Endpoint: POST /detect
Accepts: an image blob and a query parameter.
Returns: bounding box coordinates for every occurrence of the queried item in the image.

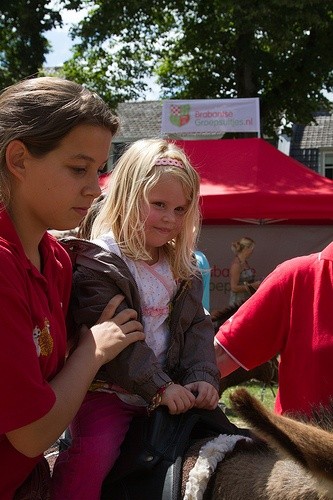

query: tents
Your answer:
[96,139,333,227]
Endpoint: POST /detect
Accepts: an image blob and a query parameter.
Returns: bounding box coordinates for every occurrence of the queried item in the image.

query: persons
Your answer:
[230,237,261,305]
[213,241,333,433]
[51,137,220,499]
[0,77,145,500]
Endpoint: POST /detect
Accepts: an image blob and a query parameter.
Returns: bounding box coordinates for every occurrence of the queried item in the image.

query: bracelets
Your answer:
[148,380,172,412]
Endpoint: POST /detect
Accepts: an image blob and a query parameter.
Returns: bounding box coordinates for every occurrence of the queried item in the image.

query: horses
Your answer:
[98,385,333,499]
